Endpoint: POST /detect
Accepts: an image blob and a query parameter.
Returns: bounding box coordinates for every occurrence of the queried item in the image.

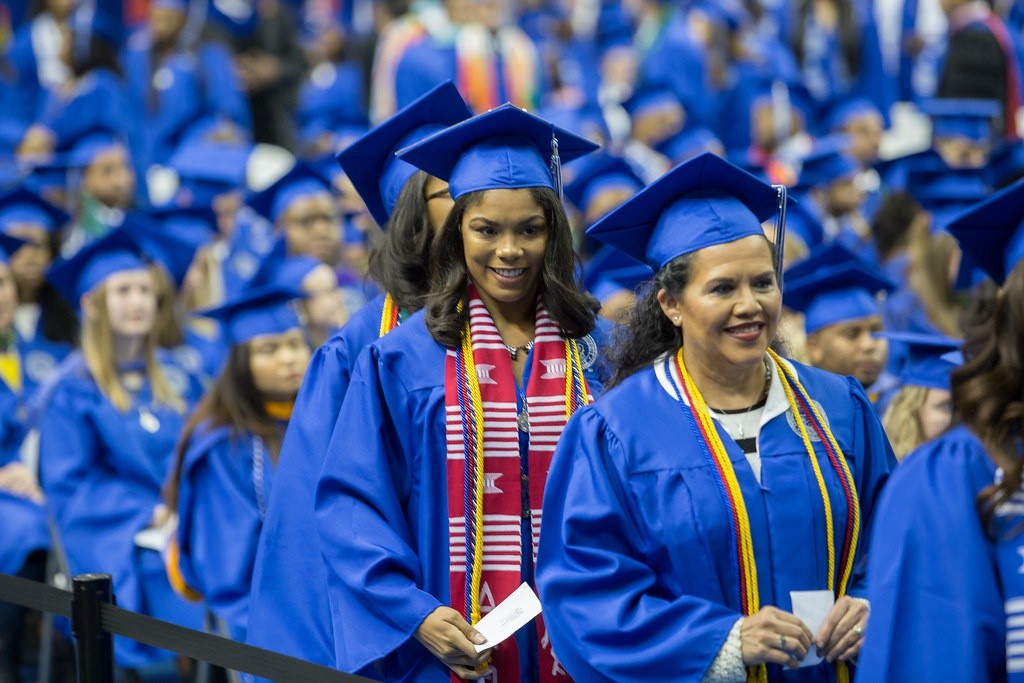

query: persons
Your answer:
[781,262,897,387]
[41,230,204,683]
[856,179,1023,683]
[534,151,897,683]
[245,160,347,261]
[870,328,969,460]
[0,0,1023,581]
[173,279,315,645]
[315,102,640,683]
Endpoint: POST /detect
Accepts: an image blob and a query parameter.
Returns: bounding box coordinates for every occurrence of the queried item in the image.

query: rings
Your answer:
[854,626,864,637]
[780,634,784,648]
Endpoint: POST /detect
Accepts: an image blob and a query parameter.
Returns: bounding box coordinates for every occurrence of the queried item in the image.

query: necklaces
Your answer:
[765,361,772,395]
[507,340,535,359]
[711,380,765,439]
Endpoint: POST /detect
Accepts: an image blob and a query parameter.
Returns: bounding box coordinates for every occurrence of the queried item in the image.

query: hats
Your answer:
[240,153,345,226]
[870,332,965,390]
[562,154,646,210]
[193,284,312,345]
[780,146,851,190]
[780,242,899,332]
[944,177,1024,287]
[585,152,797,287]
[395,103,599,204]
[45,226,151,322]
[763,192,824,258]
[246,235,325,301]
[915,97,1000,138]
[335,78,482,232]
